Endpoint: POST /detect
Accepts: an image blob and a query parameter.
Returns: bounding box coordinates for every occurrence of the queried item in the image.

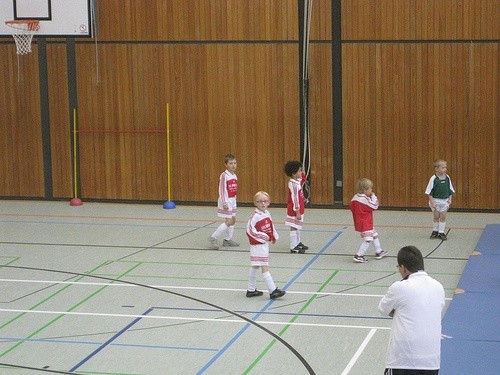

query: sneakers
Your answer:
[222,239,240,246]
[207,237,218,250]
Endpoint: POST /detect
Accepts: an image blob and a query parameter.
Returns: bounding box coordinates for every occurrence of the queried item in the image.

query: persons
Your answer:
[425,159,455,240]
[208,154,240,250]
[348,178,389,263]
[245,191,286,300]
[378,246,445,375]
[284,160,308,254]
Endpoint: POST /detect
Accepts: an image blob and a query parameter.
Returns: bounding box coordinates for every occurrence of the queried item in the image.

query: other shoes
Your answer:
[270,289,285,299]
[246,289,263,297]
[290,243,309,255]
[375,250,389,258]
[354,254,366,261]
[429,230,447,241]
